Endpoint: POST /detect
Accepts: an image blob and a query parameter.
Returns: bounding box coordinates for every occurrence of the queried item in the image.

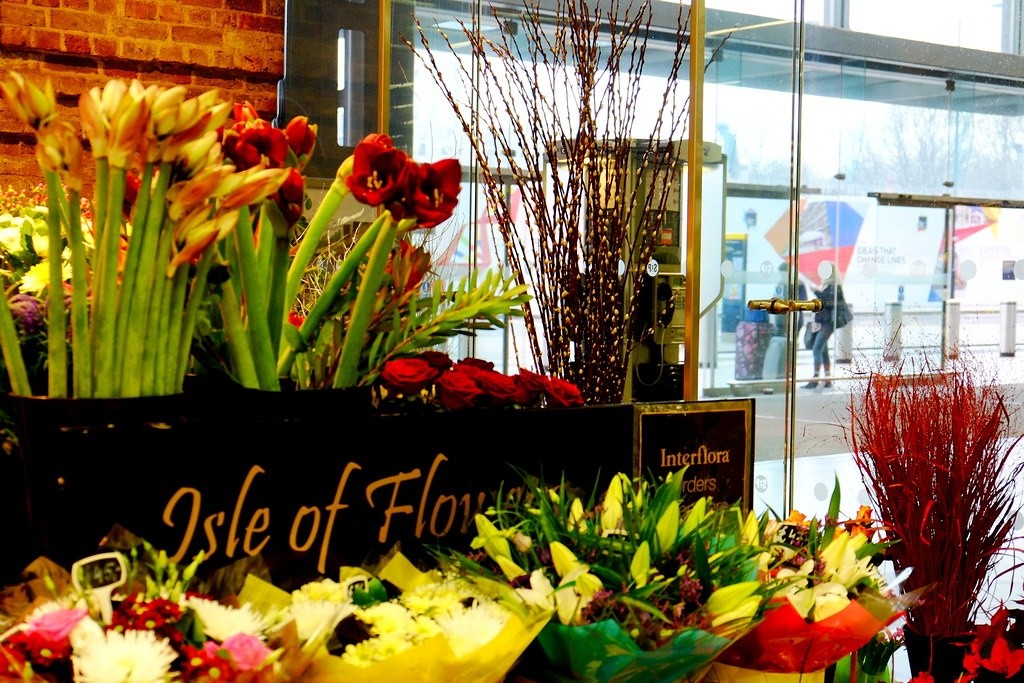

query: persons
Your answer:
[761,261,852,388]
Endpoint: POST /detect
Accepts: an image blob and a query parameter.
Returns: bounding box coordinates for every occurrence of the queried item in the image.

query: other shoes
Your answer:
[801,377,819,389]
[823,381,832,389]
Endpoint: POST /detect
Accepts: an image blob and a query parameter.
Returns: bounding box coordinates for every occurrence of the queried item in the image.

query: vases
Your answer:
[14,386,195,431]
[214,363,374,418]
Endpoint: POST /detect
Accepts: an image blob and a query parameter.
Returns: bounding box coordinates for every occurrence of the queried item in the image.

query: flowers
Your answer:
[1,1,1024,683]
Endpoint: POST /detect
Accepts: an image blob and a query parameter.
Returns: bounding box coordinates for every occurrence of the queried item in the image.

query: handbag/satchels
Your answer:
[830,285,854,328]
